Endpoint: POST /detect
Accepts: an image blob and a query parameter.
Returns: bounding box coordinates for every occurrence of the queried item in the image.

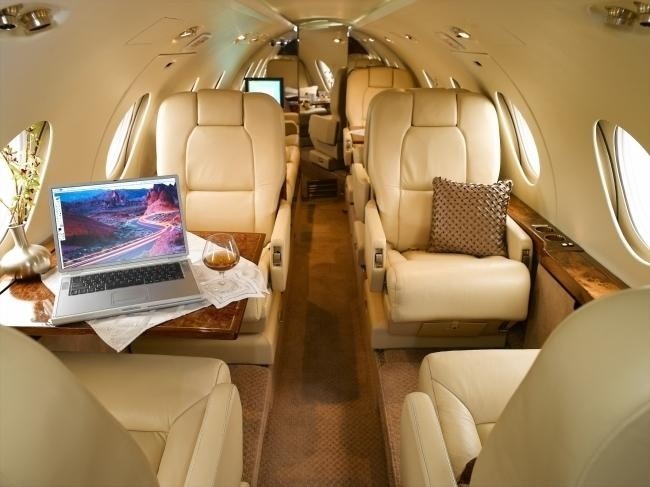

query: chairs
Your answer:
[1,325,250,487]
[358,89,535,351]
[283,112,300,198]
[308,65,359,170]
[351,88,456,269]
[342,66,415,166]
[131,88,292,367]
[396,285,650,486]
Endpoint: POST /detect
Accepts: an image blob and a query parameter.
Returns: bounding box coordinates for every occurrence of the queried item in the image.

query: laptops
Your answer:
[48,174,205,327]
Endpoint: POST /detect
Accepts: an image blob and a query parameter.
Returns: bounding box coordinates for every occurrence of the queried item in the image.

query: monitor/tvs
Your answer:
[245,77,284,108]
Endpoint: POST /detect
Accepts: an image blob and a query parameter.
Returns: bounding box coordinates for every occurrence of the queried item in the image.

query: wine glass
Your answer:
[201,234,240,293]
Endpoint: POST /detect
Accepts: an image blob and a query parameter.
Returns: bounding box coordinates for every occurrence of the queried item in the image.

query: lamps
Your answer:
[598,0,650,30]
[0,3,52,32]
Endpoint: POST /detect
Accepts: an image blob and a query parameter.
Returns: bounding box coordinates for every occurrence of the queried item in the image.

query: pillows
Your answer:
[429,177,513,260]
